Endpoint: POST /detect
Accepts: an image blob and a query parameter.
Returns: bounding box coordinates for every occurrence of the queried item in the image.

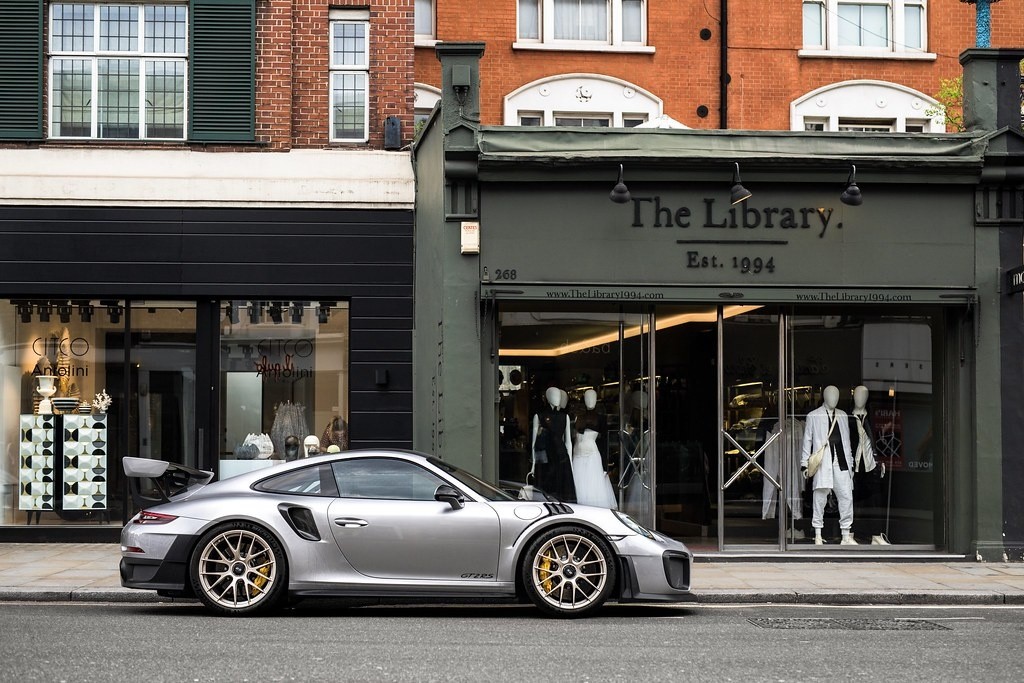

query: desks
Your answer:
[218,458,287,481]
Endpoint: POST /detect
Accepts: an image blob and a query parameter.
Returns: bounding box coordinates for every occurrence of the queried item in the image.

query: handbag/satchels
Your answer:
[808,443,825,478]
[518,472,535,501]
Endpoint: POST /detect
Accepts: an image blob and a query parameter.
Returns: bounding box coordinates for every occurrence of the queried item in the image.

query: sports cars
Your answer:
[118,446,696,616]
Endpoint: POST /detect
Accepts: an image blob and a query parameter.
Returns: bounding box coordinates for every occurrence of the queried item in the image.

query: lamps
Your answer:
[289,301,311,324]
[29,298,53,322]
[100,299,124,323]
[266,301,290,324]
[730,161,753,206]
[610,164,631,204]
[70,299,94,322]
[10,299,34,323]
[316,300,338,324]
[246,301,269,325]
[226,300,247,325]
[49,298,72,324]
[839,164,863,206]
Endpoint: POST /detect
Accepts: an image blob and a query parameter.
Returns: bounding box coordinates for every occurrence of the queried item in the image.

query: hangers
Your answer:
[772,411,806,430]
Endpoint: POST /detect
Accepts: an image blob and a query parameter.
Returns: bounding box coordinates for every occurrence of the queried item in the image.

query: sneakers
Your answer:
[871,533,891,546]
[787,528,806,539]
[813,538,827,545]
[841,532,859,546]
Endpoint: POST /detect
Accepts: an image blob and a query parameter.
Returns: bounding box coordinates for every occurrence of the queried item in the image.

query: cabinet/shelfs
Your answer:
[18,413,107,522]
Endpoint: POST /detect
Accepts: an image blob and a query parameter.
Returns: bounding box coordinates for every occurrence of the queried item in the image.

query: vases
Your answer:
[50,397,92,415]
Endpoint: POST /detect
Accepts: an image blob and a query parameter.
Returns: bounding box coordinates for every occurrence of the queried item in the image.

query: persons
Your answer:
[799,386,891,547]
[320,416,348,453]
[530,385,618,511]
